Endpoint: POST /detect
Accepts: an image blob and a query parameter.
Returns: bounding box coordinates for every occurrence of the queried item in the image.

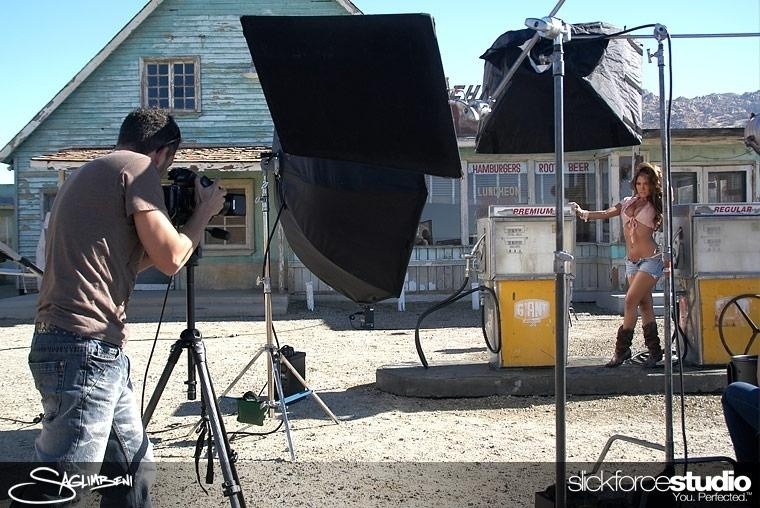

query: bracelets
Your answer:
[579,209,589,223]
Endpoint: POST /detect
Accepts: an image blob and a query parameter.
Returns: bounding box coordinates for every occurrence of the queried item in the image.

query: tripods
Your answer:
[142,245,246,508]
[186,170,340,458]
[580,39,736,480]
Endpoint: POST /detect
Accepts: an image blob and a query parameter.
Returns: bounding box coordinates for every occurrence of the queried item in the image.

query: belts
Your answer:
[35,323,76,335]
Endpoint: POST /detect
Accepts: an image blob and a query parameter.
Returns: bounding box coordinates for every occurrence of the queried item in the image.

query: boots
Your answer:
[642,321,662,367]
[605,325,634,367]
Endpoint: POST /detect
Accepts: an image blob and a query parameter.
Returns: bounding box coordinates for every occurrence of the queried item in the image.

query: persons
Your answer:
[417,226,433,246]
[721,381,760,491]
[25,107,228,508]
[568,163,667,366]
